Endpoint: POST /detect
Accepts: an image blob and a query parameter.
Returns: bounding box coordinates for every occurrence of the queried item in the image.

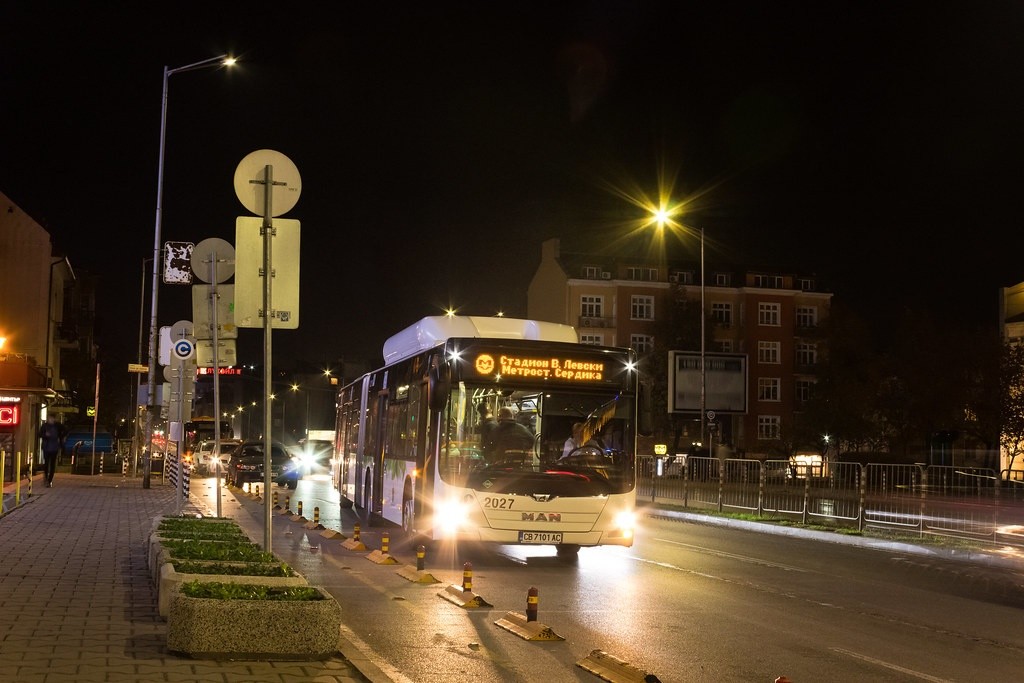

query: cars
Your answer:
[194,440,221,471]
[207,438,241,472]
[227,440,298,490]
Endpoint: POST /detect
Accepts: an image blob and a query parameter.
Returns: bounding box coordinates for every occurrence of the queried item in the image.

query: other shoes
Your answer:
[47,481,53,487]
[43,477,47,485]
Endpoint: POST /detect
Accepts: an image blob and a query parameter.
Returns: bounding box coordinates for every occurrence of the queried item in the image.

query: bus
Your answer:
[330,312,643,563]
[182,416,234,468]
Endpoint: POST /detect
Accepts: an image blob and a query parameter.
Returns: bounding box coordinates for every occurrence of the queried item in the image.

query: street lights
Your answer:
[653,211,710,455]
[146,47,242,409]
[220,366,338,444]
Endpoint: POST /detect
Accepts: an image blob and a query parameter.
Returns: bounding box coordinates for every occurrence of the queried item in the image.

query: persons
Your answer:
[562,421,605,460]
[464,402,536,465]
[39,413,69,488]
